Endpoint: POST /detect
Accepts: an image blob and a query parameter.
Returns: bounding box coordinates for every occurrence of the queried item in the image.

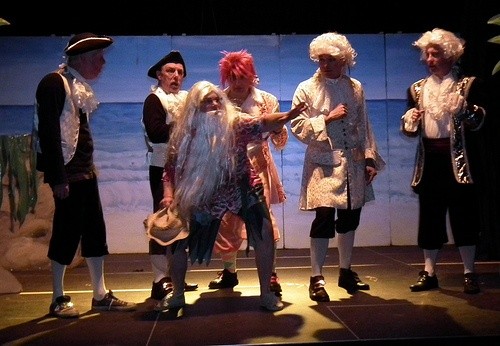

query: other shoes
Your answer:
[151,290,186,312]
[208,267,238,289]
[260,292,284,312]
[151,276,198,300]
[269,272,282,292]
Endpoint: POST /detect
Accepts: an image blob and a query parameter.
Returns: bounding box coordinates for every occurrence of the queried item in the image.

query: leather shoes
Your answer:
[337,267,370,290]
[308,275,329,300]
[464,269,478,296]
[409,271,439,291]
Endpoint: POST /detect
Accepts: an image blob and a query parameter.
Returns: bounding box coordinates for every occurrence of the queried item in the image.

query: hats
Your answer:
[64,31,113,56]
[143,205,191,247]
[148,50,188,80]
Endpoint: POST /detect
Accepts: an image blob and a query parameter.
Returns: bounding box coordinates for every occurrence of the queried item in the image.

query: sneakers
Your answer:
[91,290,137,311]
[49,295,79,319]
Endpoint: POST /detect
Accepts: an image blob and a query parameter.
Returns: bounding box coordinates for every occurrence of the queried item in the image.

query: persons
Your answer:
[290,33,386,301]
[142,51,197,300]
[153,80,309,309]
[401,30,488,293]
[209,51,288,295]
[35,34,137,317]
